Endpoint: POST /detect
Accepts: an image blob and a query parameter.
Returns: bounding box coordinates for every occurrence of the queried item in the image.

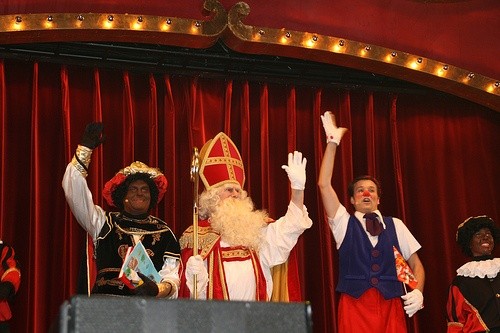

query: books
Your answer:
[119,246,135,288]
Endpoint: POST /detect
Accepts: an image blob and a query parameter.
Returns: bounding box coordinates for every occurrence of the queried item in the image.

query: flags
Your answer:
[392,246,418,289]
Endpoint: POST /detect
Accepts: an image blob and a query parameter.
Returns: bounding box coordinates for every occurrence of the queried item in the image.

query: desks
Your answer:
[61,296,313,333]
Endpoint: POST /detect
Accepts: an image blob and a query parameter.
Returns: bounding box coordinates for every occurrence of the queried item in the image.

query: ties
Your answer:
[363,212,382,237]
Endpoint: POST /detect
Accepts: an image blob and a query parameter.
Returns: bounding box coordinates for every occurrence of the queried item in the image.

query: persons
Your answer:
[62,121,184,299]
[0,238,21,333]
[441,215,500,333]
[178,132,313,304]
[318,111,425,333]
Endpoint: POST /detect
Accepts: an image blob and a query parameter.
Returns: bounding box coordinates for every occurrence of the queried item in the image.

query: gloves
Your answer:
[186,255,206,281]
[400,289,424,318]
[127,272,159,298]
[281,151,307,190]
[321,111,347,146]
[82,122,106,149]
[0,282,15,302]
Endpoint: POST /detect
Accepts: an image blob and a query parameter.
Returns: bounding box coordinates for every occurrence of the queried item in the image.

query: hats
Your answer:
[102,161,168,208]
[455,214,499,257]
[196,131,247,199]
